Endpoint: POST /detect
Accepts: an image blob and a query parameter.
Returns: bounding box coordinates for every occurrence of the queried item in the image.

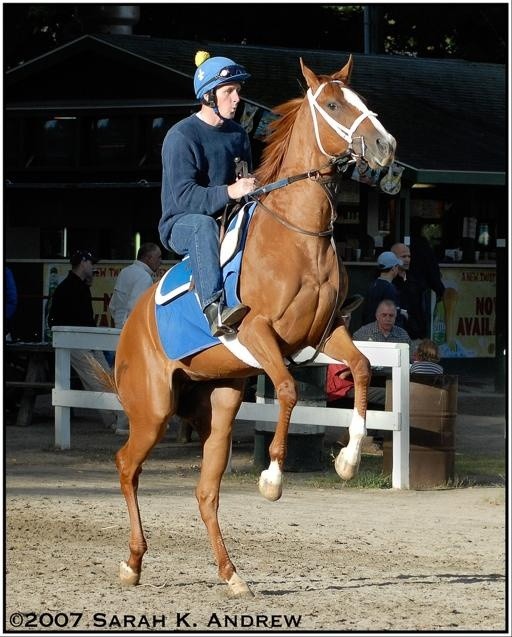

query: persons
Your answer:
[43,242,169,435]
[323,233,447,462]
[5,265,18,322]
[157,53,264,342]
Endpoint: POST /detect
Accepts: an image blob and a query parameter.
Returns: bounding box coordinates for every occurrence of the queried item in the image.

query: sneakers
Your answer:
[115,429,129,435]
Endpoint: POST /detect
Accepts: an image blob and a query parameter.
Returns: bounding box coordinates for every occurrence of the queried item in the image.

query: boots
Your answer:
[341,295,363,316]
[204,302,248,337]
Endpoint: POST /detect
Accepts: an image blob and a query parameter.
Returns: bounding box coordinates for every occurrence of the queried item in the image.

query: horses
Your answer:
[71,53,398,599]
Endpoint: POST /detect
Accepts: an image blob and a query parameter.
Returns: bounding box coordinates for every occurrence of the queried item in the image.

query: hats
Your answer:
[378,252,403,269]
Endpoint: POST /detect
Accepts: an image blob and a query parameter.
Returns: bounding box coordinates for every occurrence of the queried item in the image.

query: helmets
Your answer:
[194,51,250,99]
